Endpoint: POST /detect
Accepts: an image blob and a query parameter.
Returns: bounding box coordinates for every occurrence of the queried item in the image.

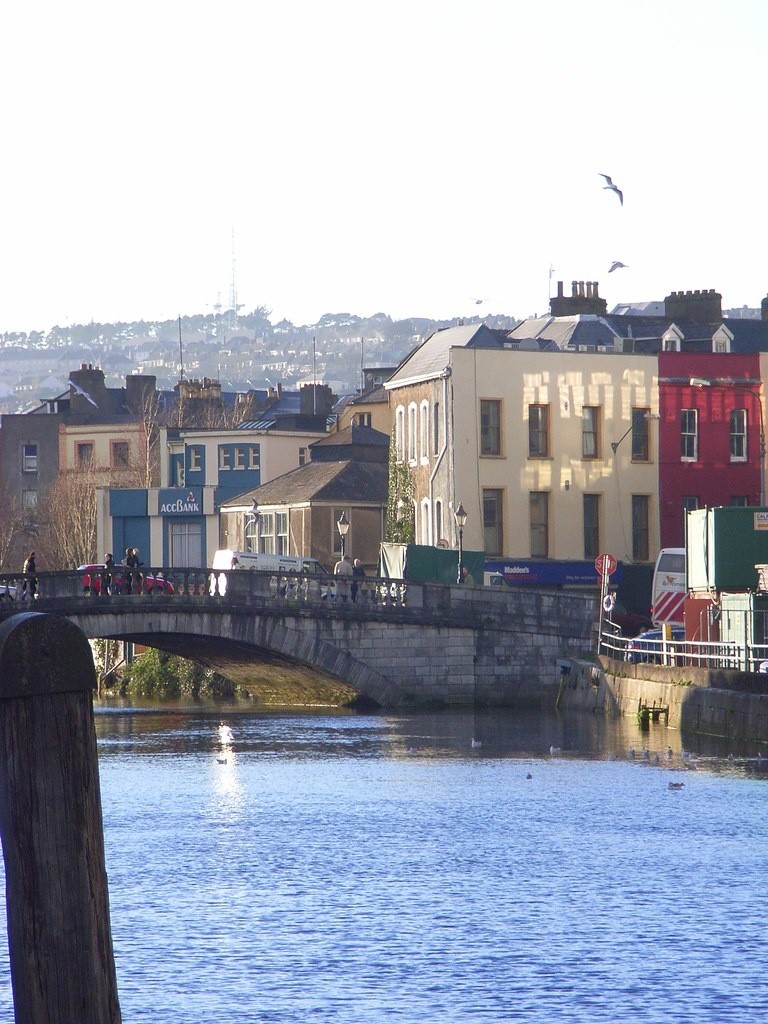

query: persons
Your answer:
[122,548,146,595]
[102,554,120,595]
[463,567,474,585]
[351,559,366,602]
[20,552,37,600]
[333,555,353,600]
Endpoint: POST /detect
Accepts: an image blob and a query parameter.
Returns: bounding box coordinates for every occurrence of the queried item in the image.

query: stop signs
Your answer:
[595,553,618,577]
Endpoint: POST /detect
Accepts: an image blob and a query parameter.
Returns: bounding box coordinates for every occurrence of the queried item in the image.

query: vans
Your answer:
[210,548,337,597]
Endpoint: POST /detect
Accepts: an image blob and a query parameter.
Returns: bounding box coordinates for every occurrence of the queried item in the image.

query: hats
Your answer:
[342,556,350,560]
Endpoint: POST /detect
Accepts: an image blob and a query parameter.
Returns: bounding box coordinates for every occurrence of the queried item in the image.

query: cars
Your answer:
[0,585,27,599]
[72,565,175,595]
[623,629,686,667]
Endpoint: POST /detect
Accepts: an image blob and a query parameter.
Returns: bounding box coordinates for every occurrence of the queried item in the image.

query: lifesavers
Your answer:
[602,596,615,613]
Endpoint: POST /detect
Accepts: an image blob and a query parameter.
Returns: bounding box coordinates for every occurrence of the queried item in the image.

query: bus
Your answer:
[648,548,689,629]
[484,555,624,594]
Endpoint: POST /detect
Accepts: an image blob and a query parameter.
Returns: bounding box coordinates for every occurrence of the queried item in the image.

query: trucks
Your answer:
[378,541,519,607]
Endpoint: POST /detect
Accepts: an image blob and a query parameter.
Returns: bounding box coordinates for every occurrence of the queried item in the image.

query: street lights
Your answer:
[453,502,468,584]
[337,511,350,562]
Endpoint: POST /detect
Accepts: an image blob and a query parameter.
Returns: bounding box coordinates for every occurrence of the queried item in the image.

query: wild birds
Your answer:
[526,771,532,779]
[470,737,482,748]
[607,745,765,791]
[549,745,563,756]
[608,260,625,273]
[217,758,228,764]
[600,173,623,205]
